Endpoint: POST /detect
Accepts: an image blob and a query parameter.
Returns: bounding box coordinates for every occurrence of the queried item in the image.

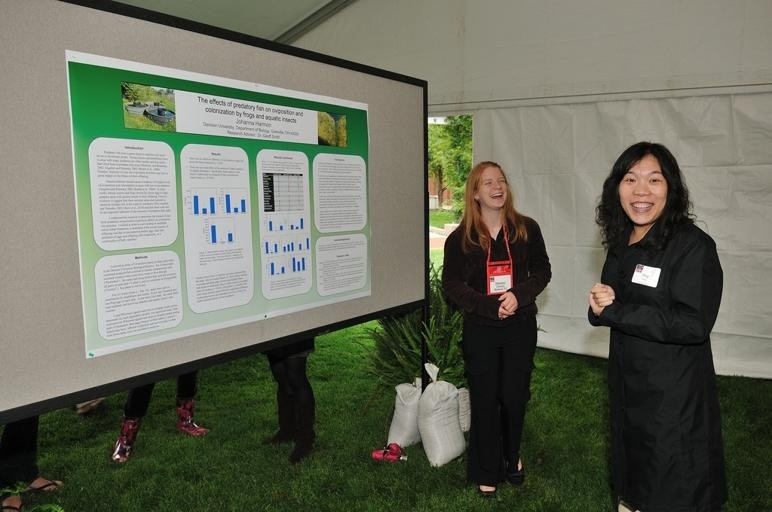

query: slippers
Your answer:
[25,476,66,493]
[0,488,26,512]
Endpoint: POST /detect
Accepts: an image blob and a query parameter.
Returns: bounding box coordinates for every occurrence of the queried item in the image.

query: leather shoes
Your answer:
[477,480,498,497]
[508,457,525,486]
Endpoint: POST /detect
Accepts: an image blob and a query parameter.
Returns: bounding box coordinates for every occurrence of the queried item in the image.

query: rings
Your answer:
[509,304,512,307]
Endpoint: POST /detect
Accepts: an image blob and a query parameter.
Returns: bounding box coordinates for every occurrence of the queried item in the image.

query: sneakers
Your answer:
[289,443,313,465]
[262,430,290,444]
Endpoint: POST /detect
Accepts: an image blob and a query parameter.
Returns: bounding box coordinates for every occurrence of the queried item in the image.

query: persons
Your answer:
[442,161,552,498]
[0,415,65,512]
[111,370,211,463]
[262,339,316,465]
[587,140,729,511]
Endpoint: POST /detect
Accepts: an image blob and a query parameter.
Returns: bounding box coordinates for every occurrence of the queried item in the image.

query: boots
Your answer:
[111,413,142,462]
[176,397,211,436]
